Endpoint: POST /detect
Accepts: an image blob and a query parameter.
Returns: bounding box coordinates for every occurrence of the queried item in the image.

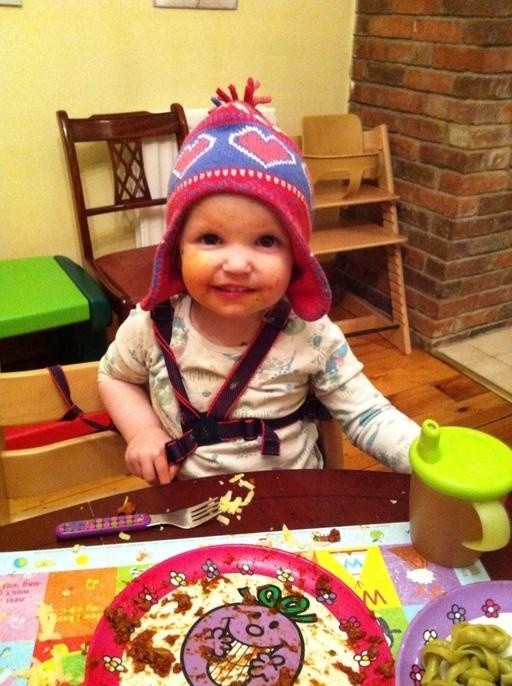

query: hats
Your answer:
[140,73,334,323]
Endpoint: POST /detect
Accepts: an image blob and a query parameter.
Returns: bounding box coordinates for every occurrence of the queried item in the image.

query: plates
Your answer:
[397,579,512,685]
[84,543,396,685]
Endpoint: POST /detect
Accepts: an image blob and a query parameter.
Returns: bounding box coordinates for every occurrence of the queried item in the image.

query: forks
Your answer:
[54,495,221,541]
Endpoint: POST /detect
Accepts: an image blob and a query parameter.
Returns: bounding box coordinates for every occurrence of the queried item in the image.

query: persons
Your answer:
[94,91,424,481]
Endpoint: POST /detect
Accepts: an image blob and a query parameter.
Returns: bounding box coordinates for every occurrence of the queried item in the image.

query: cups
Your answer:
[406,413,512,571]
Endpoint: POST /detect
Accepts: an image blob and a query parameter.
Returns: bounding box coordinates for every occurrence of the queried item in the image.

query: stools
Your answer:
[0,256,112,375]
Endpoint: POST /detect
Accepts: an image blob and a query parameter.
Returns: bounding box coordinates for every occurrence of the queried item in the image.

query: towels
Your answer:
[133,102,280,250]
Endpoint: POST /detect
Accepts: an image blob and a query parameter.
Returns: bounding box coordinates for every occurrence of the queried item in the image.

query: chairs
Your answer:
[268,113,412,356]
[58,103,192,349]
[0,363,345,526]
